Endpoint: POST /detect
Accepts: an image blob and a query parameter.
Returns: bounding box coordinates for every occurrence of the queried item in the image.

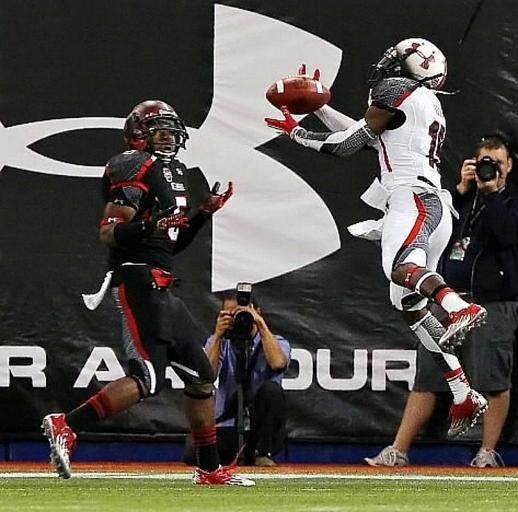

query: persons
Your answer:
[363,130,518,468]
[41,100,256,486]
[184,290,291,467]
[264,37,489,438]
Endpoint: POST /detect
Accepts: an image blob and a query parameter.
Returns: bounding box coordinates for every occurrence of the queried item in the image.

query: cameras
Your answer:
[468,156,502,181]
[221,282,254,338]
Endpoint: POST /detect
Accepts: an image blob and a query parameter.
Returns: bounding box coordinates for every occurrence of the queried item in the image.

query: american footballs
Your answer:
[267,76,332,114]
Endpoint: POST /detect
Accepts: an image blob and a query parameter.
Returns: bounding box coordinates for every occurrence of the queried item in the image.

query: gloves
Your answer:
[199,181,234,214]
[264,105,299,137]
[147,206,189,240]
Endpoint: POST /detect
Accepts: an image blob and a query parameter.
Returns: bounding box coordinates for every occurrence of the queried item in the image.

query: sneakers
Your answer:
[437,303,487,354]
[40,412,76,480]
[191,463,256,488]
[468,448,500,469]
[364,446,409,468]
[255,456,277,467]
[447,390,488,440]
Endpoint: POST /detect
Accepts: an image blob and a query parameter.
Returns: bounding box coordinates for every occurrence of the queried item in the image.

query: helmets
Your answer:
[369,36,450,90]
[123,98,189,160]
